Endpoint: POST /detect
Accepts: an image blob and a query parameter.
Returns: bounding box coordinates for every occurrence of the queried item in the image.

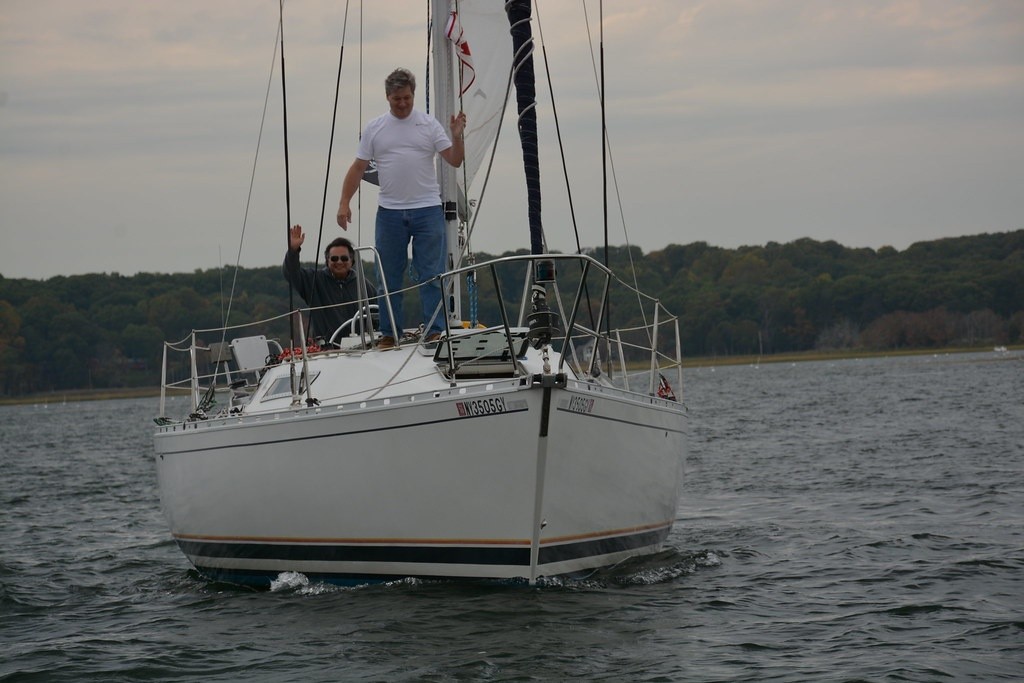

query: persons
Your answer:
[283,224,380,351]
[337,67,467,348]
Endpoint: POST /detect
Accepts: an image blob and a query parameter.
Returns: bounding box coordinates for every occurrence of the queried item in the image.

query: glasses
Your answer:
[328,255,350,262]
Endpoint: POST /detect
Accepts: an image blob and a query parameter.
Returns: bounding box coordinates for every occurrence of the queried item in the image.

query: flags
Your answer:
[657,374,676,401]
[445,10,478,100]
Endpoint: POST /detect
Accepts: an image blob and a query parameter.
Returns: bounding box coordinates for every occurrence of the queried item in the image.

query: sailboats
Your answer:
[154,2,686,587]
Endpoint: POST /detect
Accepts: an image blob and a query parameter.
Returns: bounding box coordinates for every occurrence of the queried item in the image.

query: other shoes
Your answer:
[424,333,441,344]
[377,336,395,348]
